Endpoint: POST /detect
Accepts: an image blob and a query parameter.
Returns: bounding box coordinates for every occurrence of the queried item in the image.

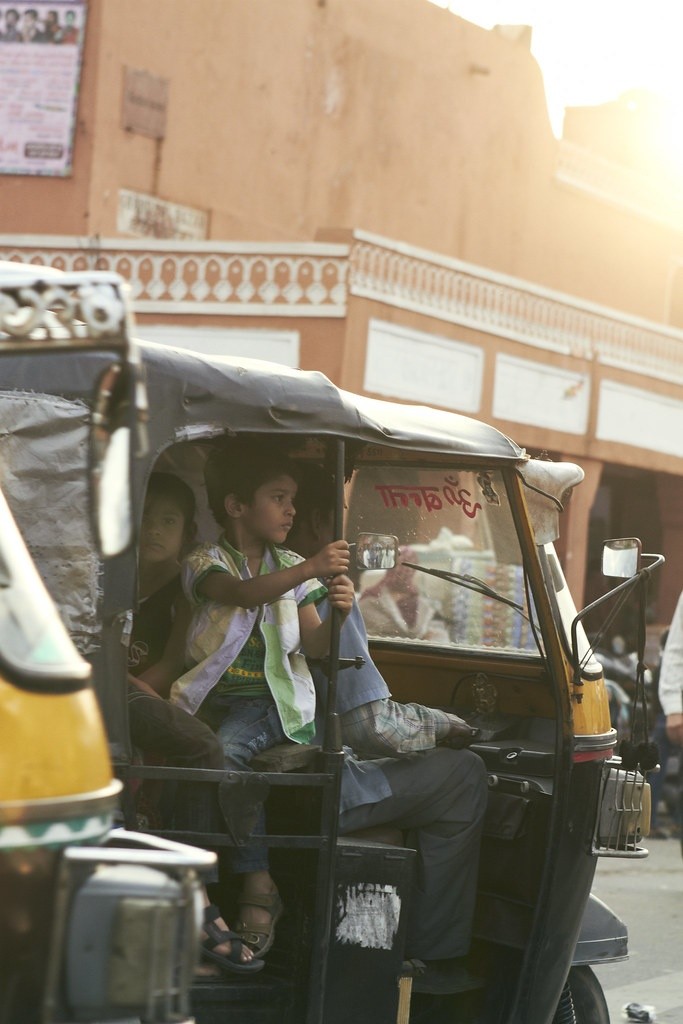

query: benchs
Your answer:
[260,744,404,848]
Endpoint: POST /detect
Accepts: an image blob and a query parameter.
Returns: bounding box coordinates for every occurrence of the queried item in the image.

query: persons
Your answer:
[170,442,355,959]
[658,590,683,746]
[125,471,265,976]
[277,463,489,994]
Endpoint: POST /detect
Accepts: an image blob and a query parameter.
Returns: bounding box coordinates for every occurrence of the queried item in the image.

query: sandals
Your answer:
[232,884,282,956]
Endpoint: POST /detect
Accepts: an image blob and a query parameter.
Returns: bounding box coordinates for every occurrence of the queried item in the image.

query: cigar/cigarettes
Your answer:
[348,543,357,546]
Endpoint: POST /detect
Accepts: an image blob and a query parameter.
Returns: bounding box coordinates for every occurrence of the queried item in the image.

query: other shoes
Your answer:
[647,830,667,839]
[412,959,478,995]
[671,829,683,838]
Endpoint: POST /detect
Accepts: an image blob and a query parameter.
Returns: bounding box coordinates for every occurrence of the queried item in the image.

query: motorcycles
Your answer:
[0,490,219,1024]
[0,258,666,1024]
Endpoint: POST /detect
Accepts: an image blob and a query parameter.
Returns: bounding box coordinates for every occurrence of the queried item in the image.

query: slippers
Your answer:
[191,904,266,975]
